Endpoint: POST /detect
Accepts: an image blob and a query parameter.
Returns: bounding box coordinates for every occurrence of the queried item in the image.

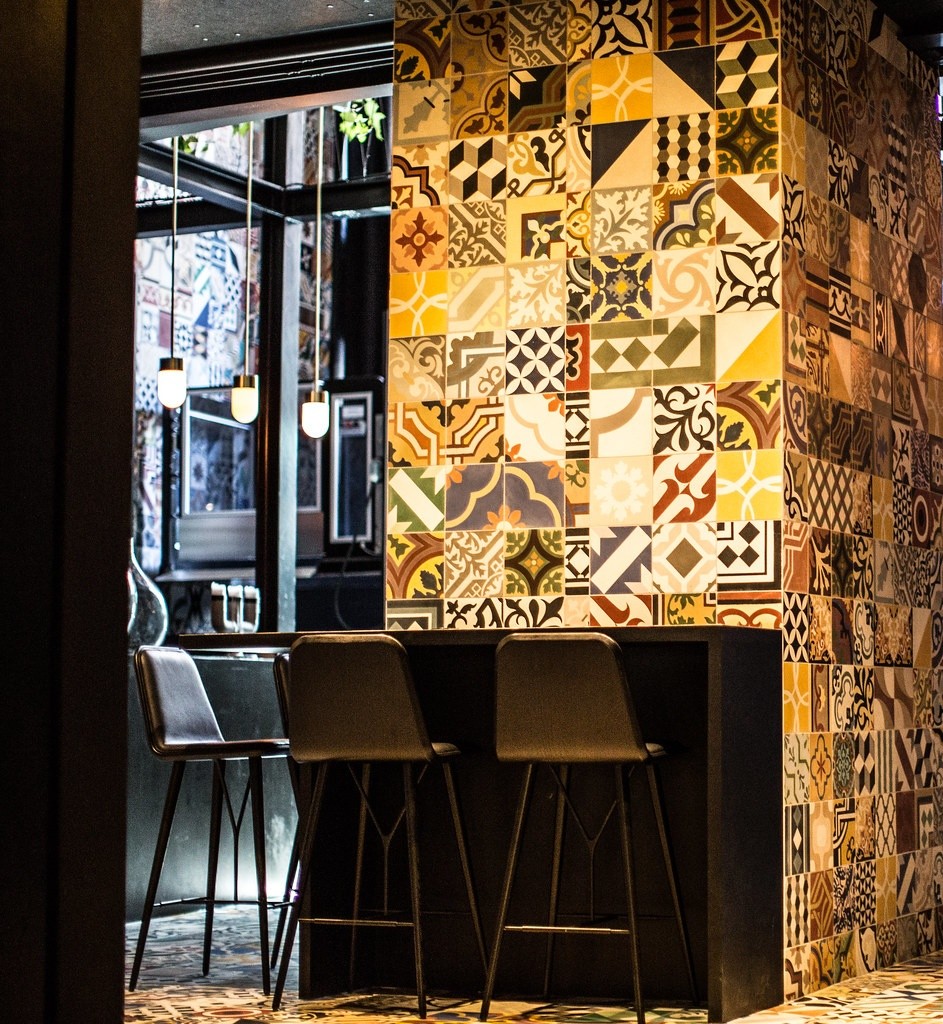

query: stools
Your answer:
[272,635,489,1018]
[271,651,302,969]
[128,644,291,995]
[478,632,700,1024]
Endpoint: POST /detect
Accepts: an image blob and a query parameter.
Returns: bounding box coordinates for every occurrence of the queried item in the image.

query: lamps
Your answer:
[157,136,187,410]
[302,105,329,438]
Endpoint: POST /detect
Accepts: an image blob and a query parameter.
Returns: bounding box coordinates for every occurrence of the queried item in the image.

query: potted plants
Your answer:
[230,121,258,423]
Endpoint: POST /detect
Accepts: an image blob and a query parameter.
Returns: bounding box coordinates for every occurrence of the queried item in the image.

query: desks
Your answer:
[177,627,784,1024]
